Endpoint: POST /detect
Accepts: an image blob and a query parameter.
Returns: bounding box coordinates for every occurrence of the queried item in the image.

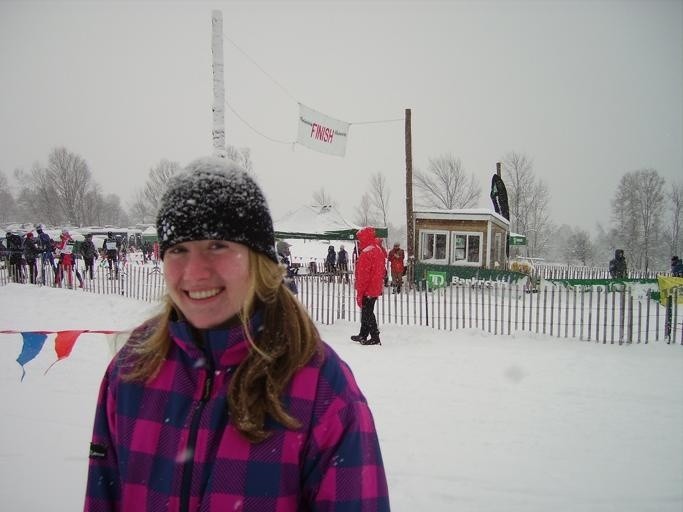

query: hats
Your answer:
[276,240,292,252]
[27,233,33,238]
[157,158,278,263]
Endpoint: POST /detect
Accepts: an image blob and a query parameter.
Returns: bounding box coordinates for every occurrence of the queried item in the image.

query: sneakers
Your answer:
[351,336,367,341]
[362,338,381,345]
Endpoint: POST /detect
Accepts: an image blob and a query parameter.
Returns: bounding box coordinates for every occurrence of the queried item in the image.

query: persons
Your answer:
[0,224,152,288]
[81,155,393,510]
[349,227,387,346]
[670,255,682,279]
[389,242,406,294]
[336,244,350,283]
[324,245,337,273]
[609,248,631,280]
[276,241,300,296]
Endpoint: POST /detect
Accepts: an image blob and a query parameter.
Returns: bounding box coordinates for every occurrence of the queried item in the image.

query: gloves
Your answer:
[357,294,363,306]
